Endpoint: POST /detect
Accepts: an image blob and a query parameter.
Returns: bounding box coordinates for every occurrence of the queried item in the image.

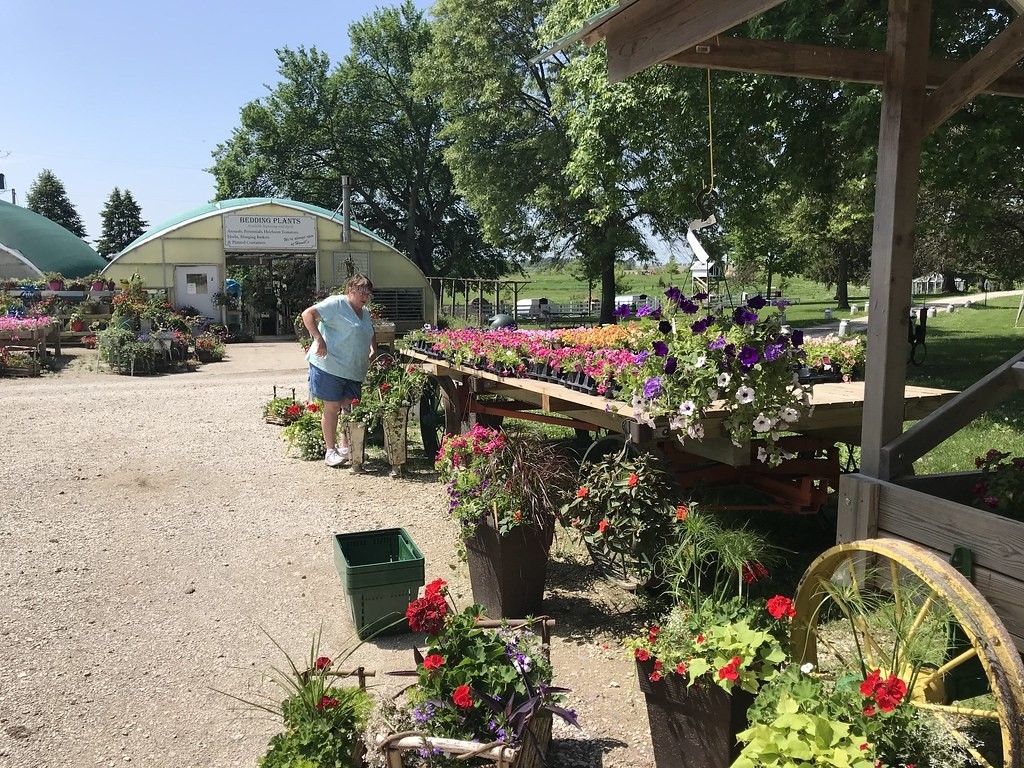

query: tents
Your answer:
[0,198,437,336]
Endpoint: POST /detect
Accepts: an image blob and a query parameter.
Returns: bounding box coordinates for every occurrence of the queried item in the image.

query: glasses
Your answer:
[352,287,369,298]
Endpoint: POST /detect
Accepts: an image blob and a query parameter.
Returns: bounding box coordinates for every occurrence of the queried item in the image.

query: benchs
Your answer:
[543,312,594,328]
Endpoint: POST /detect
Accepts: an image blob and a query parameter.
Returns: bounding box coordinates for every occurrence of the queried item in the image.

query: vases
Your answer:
[583,534,673,590]
[0,364,40,377]
[0,324,54,339]
[50,281,62,290]
[93,281,103,291]
[72,321,83,331]
[462,519,555,618]
[636,652,763,768]
[380,619,554,768]
[345,422,368,475]
[382,406,409,478]
[196,350,220,362]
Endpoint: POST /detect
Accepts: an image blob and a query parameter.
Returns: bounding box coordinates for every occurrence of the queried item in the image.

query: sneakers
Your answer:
[337,447,352,465]
[325,449,346,466]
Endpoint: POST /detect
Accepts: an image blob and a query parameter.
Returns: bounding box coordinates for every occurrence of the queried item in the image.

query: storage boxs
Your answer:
[332,527,425,640]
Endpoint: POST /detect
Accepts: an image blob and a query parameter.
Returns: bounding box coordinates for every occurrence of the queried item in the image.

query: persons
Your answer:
[302,275,378,467]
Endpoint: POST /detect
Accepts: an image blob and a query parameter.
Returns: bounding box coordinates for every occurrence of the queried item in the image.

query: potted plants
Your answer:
[265,398,303,426]
[120,279,129,291]
[212,293,237,310]
[468,393,507,432]
[116,342,155,374]
[108,278,115,290]
[186,360,197,372]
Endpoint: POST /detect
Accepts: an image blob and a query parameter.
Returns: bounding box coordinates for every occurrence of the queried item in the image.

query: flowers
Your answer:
[0,270,224,369]
[255,288,908,768]
